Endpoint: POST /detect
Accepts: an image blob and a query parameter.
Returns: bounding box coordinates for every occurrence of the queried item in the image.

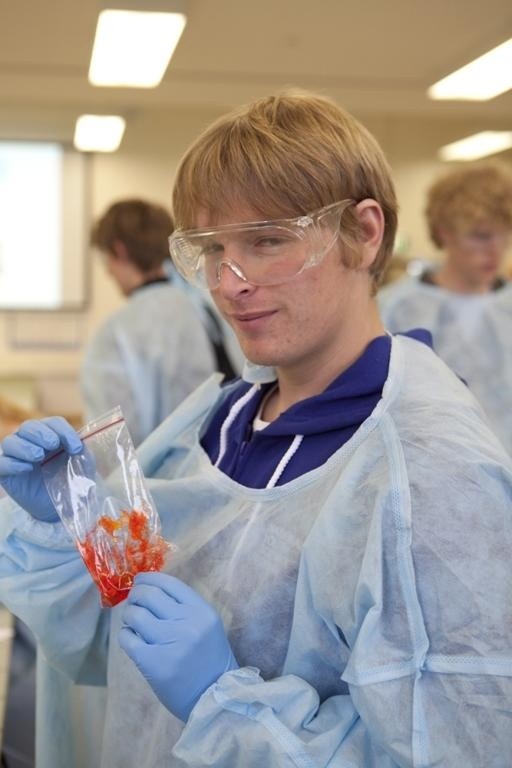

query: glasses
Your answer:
[167,198,360,292]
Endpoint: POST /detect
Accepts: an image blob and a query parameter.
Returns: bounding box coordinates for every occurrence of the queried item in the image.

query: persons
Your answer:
[358,154,508,441]
[72,190,243,436]
[0,80,511,767]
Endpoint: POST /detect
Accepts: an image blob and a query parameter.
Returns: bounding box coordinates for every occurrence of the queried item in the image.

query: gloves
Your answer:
[0,415,99,524]
[116,572,241,726]
